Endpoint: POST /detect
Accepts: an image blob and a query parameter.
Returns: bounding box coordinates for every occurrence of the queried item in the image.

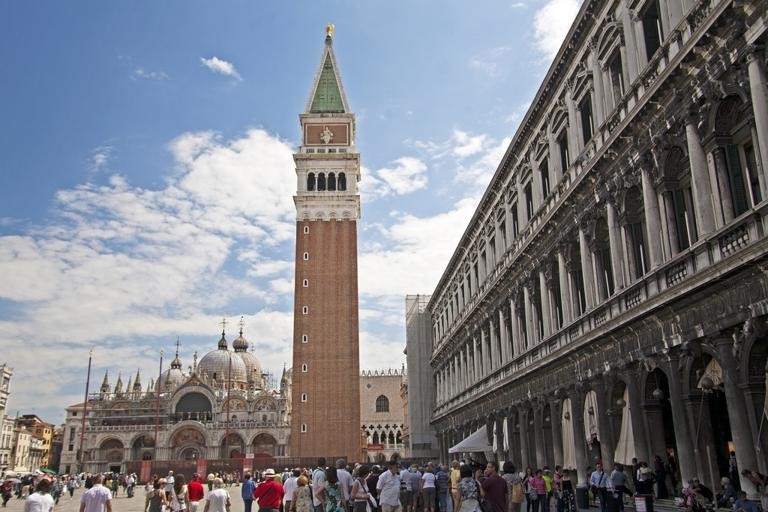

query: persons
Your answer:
[4,446,768,511]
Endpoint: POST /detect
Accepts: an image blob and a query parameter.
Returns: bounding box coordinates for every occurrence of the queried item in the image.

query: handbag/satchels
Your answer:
[480,501,491,512]
[591,485,598,494]
[511,481,524,503]
[529,489,537,500]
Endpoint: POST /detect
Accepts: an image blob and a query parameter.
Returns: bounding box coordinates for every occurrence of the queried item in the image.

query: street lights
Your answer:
[75,348,95,475]
[224,350,233,457]
[151,347,165,461]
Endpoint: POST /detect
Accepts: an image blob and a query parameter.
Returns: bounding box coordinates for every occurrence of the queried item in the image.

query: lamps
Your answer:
[477,345,713,439]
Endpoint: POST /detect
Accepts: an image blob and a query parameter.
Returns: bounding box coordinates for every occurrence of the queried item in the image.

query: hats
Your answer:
[265,468,275,477]
